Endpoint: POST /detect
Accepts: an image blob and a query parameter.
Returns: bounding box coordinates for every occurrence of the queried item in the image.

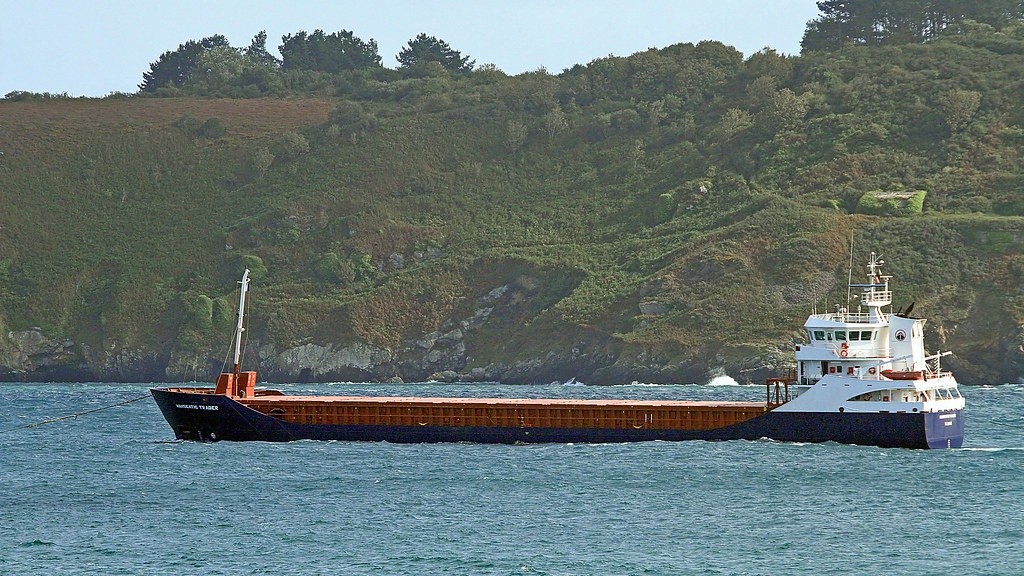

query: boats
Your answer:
[148,227,969,452]
[879,370,922,381]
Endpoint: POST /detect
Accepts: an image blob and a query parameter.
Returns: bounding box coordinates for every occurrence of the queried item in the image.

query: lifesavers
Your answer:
[869,367,875,374]
[863,394,872,401]
[840,350,848,356]
